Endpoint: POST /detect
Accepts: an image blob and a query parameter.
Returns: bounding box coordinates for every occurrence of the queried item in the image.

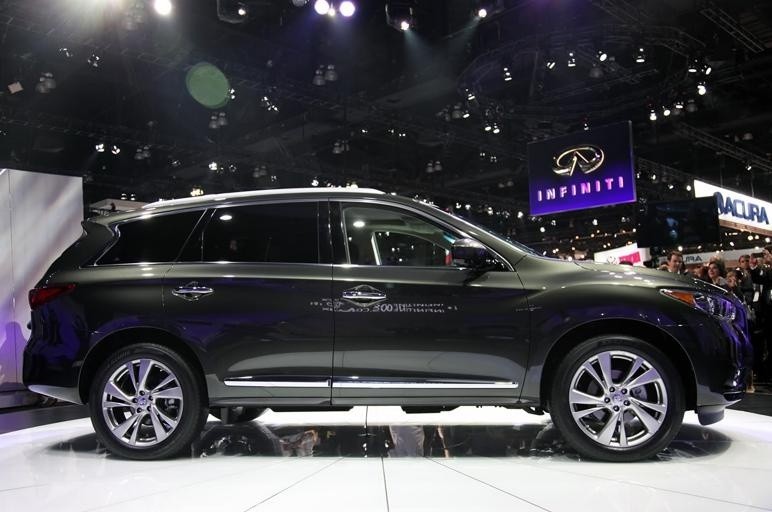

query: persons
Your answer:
[618,243,772,393]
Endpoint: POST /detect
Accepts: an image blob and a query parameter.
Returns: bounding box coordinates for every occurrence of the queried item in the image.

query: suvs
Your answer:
[23,186,752,462]
